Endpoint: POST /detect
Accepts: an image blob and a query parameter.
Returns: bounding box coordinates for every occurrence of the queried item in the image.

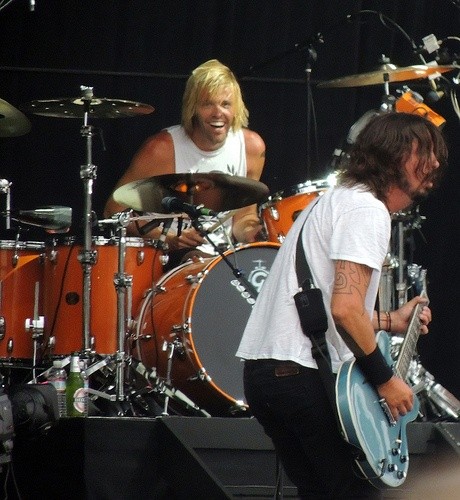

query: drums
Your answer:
[0,238,45,371]
[258,180,330,242]
[347,110,381,144]
[43,236,169,355]
[126,242,282,418]
[381,85,447,131]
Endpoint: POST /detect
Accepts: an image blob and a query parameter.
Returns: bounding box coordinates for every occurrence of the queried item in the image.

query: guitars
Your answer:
[335,269,430,493]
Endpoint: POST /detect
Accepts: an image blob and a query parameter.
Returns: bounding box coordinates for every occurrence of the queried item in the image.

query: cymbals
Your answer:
[112,173,270,215]
[0,98,32,137]
[315,64,455,89]
[17,98,156,119]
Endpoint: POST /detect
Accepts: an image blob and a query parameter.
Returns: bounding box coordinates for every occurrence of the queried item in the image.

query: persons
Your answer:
[235,107,449,500]
[105,59,266,272]
